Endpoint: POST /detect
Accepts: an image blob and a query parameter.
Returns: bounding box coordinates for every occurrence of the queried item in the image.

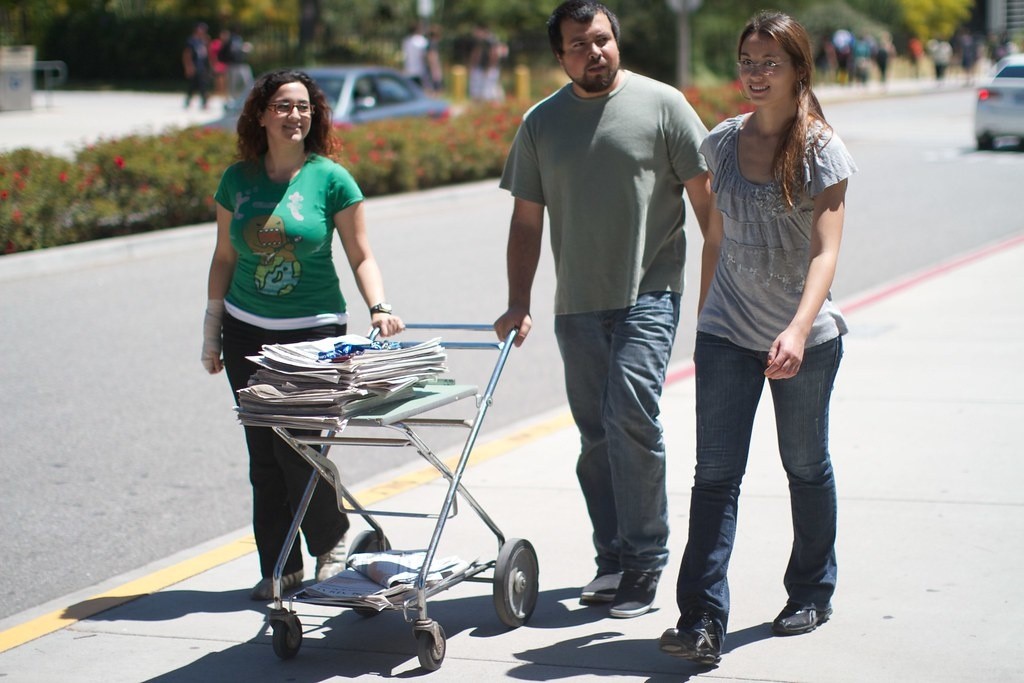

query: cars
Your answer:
[184,65,452,141]
[974,54,1024,151]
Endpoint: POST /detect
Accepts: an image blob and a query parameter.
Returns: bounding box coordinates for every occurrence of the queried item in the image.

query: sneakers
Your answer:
[253,569,304,599]
[315,533,346,583]
[772,604,833,635]
[659,609,722,665]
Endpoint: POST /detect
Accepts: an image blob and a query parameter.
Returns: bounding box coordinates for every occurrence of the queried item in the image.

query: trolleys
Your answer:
[245,322,540,672]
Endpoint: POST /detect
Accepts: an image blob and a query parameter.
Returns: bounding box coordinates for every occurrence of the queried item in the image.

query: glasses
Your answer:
[736,60,795,74]
[267,103,314,114]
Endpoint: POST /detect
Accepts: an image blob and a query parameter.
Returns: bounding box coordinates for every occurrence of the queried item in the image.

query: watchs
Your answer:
[371,304,392,314]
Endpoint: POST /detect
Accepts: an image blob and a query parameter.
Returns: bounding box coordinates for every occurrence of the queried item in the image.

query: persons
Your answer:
[201,68,404,599]
[469,24,508,100]
[908,34,922,77]
[494,0,709,615]
[184,23,253,111]
[814,31,895,84]
[660,14,857,668]
[402,20,444,90]
[924,30,1018,78]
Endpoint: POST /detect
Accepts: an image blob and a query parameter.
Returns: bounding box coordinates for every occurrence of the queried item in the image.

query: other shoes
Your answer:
[581,569,623,602]
[609,569,663,618]
